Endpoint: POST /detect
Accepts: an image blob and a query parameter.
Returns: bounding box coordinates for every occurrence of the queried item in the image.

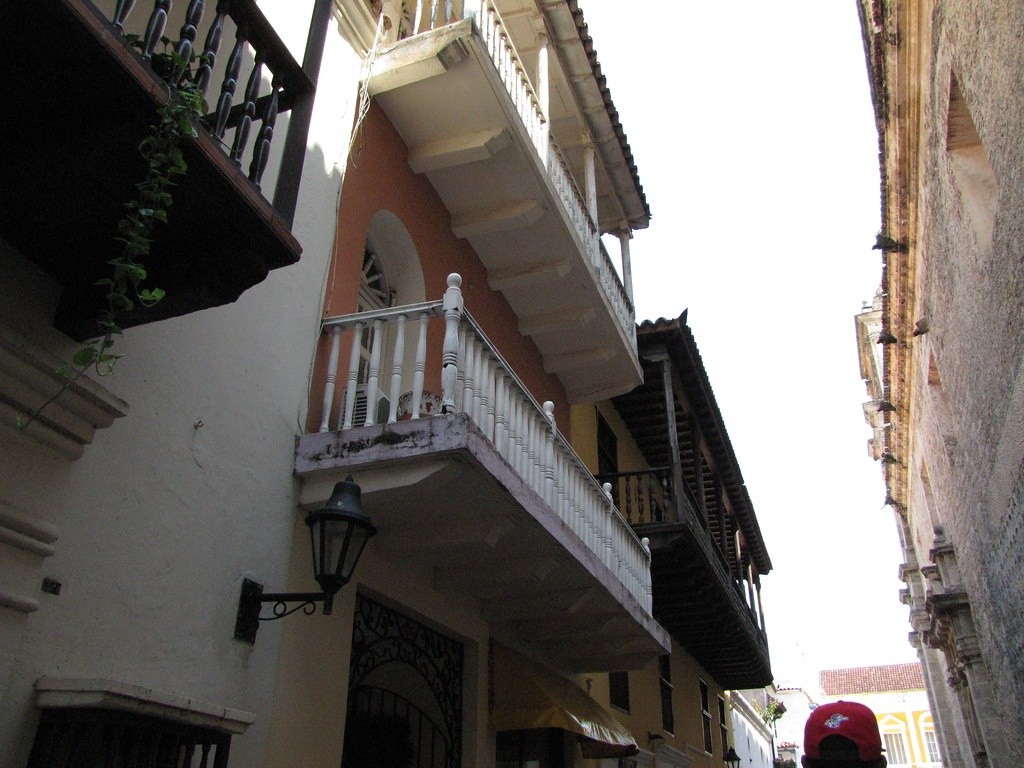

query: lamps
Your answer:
[236,473,377,645]
[648,731,666,745]
[725,747,741,768]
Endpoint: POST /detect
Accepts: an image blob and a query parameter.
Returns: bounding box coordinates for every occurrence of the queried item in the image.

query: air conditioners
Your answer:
[338,382,402,429]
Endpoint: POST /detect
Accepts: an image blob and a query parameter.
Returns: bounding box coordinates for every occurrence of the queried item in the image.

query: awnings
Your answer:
[492,646,638,755]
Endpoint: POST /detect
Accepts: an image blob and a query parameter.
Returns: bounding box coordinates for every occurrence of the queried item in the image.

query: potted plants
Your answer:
[16,33,211,431]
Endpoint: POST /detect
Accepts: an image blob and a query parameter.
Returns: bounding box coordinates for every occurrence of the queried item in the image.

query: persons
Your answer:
[801,699,887,768]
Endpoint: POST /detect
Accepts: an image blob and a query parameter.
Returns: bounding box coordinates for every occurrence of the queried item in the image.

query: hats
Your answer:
[803,700,886,761]
